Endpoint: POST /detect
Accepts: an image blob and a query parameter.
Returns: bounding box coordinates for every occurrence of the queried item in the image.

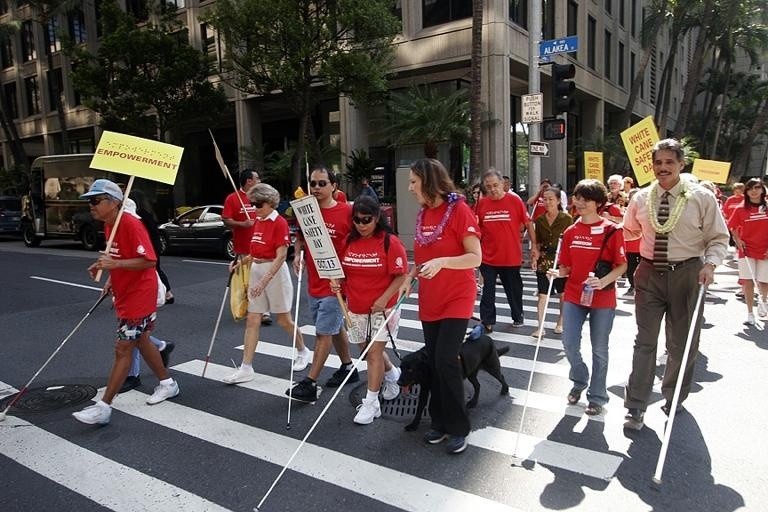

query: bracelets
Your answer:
[407,271,416,281]
[255,270,274,290]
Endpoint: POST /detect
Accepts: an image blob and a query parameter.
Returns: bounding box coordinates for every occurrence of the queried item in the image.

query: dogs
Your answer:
[397,335,511,432]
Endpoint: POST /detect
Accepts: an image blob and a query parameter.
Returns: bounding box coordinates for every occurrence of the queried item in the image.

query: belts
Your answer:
[641,256,700,272]
[252,258,273,264]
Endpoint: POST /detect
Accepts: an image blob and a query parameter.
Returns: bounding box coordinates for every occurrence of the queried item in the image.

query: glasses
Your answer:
[352,214,374,224]
[250,200,266,208]
[310,180,332,187]
[87,198,110,204]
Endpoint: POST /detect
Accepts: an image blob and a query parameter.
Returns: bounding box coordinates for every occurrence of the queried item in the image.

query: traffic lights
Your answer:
[550,64,578,116]
[542,119,566,139]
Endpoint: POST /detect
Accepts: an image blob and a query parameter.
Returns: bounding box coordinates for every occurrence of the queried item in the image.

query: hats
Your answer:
[623,176,634,184]
[77,178,124,203]
[120,198,142,220]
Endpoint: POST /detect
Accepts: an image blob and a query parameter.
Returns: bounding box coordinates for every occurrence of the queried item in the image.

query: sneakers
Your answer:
[326,368,359,387]
[757,301,768,317]
[747,312,756,326]
[164,296,175,305]
[292,351,313,371]
[353,398,382,424]
[448,431,468,454]
[482,321,493,334]
[72,401,113,426]
[622,405,645,429]
[664,399,684,415]
[735,288,759,299]
[512,312,525,328]
[222,366,254,385]
[286,380,317,402]
[145,380,179,405]
[531,328,545,340]
[259,312,273,324]
[424,428,447,444]
[382,368,401,401]
[554,323,564,334]
[621,286,637,301]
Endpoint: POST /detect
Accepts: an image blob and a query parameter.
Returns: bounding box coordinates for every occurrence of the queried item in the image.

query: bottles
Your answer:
[579,270,596,307]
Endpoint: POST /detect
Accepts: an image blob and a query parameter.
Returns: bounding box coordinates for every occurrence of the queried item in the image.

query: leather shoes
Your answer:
[112,376,141,393]
[160,339,175,370]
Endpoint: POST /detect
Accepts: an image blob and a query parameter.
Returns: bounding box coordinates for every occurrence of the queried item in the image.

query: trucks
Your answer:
[21,151,171,251]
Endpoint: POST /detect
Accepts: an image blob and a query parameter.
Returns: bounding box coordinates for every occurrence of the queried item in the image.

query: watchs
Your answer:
[704,262,716,270]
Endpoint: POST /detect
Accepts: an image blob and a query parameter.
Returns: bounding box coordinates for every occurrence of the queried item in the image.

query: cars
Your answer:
[1,194,27,239]
[156,204,301,261]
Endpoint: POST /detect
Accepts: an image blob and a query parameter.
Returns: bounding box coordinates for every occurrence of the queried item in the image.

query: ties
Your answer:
[652,192,673,277]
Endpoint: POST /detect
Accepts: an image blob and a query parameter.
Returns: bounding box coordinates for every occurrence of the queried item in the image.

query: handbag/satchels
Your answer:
[595,261,614,290]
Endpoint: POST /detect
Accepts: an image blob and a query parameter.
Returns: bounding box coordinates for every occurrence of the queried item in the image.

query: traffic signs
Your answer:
[537,35,580,57]
[527,141,551,158]
[521,93,543,122]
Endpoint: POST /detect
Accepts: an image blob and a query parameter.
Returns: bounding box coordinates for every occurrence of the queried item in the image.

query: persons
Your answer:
[355,177,379,204]
[220,168,274,326]
[222,182,314,386]
[103,182,176,394]
[328,175,349,204]
[602,175,641,301]
[399,158,484,455]
[700,178,768,326]
[615,138,733,432]
[472,170,574,338]
[71,178,180,426]
[545,177,629,416]
[283,166,360,403]
[329,194,409,426]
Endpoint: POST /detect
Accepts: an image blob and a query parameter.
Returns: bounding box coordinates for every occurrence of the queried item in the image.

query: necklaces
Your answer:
[645,178,691,235]
[414,193,459,246]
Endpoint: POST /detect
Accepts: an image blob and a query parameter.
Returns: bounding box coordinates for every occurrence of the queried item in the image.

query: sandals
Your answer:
[584,402,603,416]
[567,381,589,405]
